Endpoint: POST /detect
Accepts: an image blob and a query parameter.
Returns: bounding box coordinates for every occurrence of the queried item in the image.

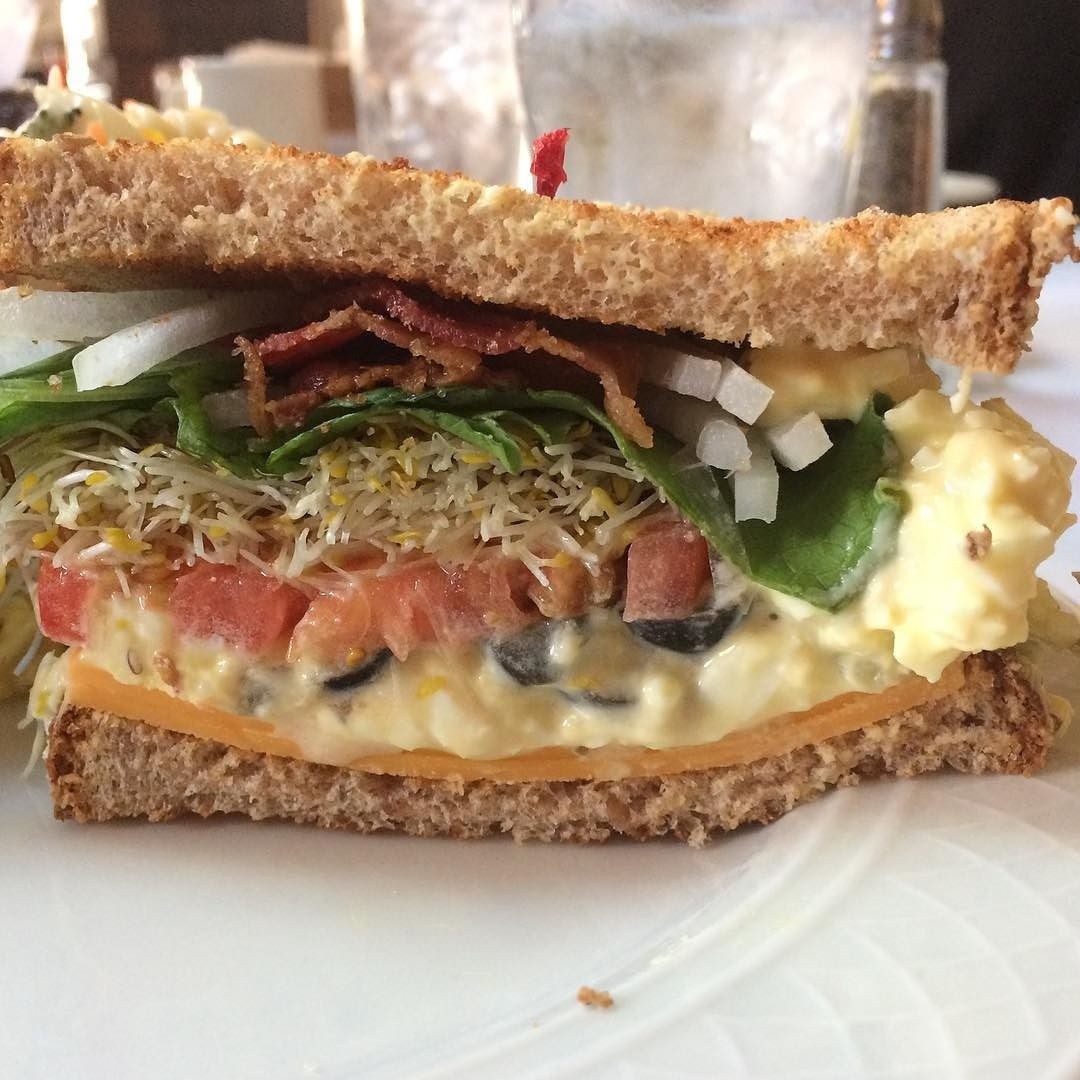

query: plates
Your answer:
[0,218,1080,1080]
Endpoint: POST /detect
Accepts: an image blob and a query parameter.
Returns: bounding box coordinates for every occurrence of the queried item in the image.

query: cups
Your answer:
[846,1,946,215]
[343,1,531,184]
[181,47,326,160]
[513,1,873,219]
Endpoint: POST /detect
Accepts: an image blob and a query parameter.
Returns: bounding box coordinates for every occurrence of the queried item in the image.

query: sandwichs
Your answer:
[1,62,1080,844]
